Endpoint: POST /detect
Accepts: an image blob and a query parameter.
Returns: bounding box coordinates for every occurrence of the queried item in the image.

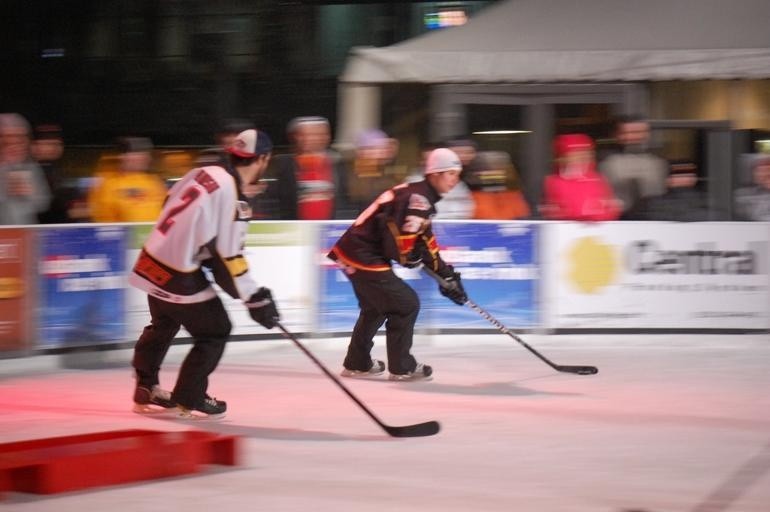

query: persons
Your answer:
[328,147,463,377]
[126,127,285,416]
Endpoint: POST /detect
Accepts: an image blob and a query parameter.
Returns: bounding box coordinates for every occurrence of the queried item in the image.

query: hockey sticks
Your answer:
[273,321,440,436]
[419,260,598,374]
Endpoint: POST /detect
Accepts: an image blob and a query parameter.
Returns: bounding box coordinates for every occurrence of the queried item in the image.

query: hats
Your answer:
[552,133,597,158]
[222,129,276,158]
[422,147,464,176]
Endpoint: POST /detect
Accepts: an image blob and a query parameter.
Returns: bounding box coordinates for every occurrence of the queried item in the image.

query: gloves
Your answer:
[436,261,468,306]
[245,286,281,330]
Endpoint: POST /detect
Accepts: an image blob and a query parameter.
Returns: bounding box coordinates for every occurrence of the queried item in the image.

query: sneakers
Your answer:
[173,387,227,414]
[344,359,385,373]
[132,381,177,410]
[388,362,432,380]
